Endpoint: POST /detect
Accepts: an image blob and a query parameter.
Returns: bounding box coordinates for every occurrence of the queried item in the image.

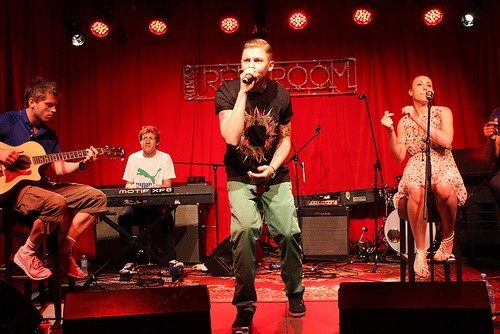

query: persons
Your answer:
[0,78,107,279]
[116,126,177,256]
[215,38,306,334]
[484,107,500,205]
[380,77,467,280]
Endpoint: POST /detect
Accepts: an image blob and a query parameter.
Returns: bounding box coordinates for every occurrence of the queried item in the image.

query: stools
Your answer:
[0,199,63,322]
[398,195,462,281]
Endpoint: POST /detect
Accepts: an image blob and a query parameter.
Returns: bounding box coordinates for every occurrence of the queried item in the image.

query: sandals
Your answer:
[414,243,430,279]
[433,232,455,262]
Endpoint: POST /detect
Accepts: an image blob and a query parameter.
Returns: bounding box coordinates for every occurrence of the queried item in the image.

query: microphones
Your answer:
[243,73,254,84]
[426,90,433,99]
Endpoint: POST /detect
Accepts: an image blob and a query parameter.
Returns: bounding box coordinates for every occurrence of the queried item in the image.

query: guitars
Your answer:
[0,140,126,201]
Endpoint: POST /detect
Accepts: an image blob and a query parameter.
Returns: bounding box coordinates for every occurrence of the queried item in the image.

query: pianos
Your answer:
[82,183,215,288]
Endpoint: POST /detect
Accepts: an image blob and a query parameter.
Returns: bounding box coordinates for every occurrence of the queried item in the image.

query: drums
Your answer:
[383,208,438,255]
[387,189,398,216]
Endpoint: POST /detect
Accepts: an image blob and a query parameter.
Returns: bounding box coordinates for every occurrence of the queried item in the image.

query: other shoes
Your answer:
[169,260,184,270]
[119,264,137,273]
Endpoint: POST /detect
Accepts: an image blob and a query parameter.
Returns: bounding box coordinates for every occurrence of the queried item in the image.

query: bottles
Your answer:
[81,255,88,276]
[487,284,496,320]
[480,273,488,285]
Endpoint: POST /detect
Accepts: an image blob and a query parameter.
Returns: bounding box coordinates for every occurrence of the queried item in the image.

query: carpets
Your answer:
[65,254,493,305]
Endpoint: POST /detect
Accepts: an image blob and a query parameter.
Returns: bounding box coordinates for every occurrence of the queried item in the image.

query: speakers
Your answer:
[338,280,494,334]
[298,206,349,260]
[61,284,211,334]
[0,277,44,334]
[205,232,265,277]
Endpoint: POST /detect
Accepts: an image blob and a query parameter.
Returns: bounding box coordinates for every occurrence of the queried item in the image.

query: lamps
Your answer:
[458,0,480,30]
[349,0,388,48]
[86,0,125,41]
[62,12,87,49]
[208,0,249,42]
[133,0,176,42]
[268,0,325,47]
[416,0,450,33]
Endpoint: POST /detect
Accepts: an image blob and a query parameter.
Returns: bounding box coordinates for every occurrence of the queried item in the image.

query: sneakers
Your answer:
[288,294,306,317]
[14,246,52,280]
[51,250,86,279]
[231,310,253,331]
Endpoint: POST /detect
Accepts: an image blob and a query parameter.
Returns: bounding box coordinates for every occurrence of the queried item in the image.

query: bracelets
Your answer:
[78,160,88,171]
[271,165,276,178]
